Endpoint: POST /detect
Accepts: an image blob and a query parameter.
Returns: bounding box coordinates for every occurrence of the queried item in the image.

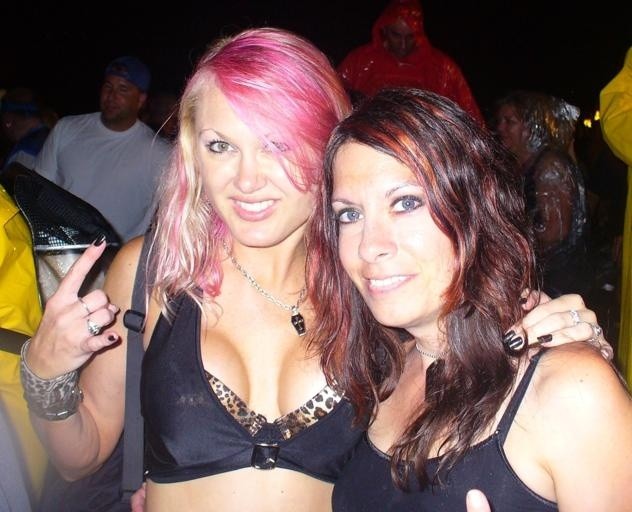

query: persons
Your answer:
[0,2,630,512]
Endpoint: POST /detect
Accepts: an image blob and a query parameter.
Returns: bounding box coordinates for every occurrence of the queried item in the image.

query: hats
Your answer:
[107,56,151,91]
